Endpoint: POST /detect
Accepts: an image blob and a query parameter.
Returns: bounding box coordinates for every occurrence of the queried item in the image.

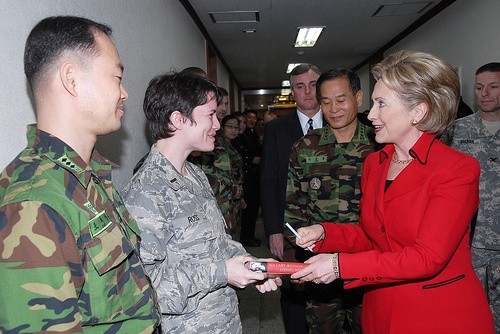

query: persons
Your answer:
[440,62,500,334]
[133,49,473,334]
[120,70,284,334]
[0,16,160,334]
[295,49,495,334]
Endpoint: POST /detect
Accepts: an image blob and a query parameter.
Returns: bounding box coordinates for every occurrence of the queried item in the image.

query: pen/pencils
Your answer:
[286,222,313,253]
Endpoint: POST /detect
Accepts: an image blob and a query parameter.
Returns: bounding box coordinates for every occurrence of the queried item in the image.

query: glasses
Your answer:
[225,125,240,131]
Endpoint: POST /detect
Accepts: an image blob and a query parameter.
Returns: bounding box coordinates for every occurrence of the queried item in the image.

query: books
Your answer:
[247,260,310,275]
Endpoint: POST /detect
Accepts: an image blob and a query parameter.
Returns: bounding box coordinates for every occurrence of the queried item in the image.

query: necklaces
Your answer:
[392,152,414,164]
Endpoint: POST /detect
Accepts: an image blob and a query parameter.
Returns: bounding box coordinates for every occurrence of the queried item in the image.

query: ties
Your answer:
[307,119,313,134]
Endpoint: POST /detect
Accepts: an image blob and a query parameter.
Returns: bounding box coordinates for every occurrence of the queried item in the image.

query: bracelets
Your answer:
[332,253,339,278]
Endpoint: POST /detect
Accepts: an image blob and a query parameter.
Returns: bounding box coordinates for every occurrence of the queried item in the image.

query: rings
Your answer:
[313,279,319,284]
